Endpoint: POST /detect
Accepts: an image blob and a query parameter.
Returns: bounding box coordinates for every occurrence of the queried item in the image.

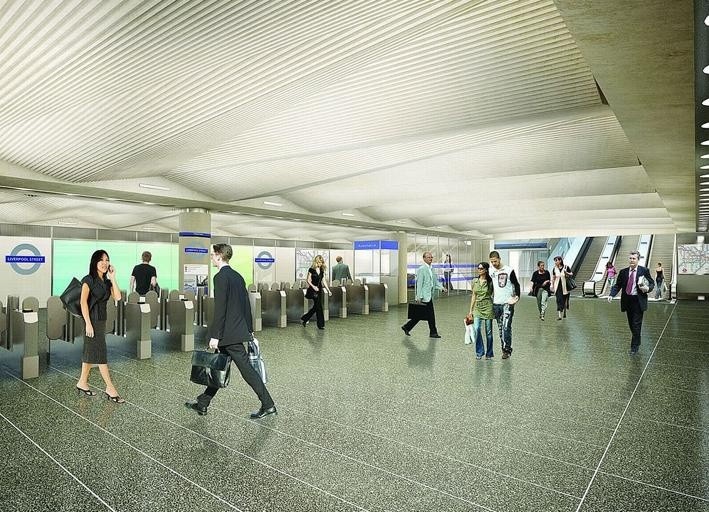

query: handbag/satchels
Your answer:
[464,318,472,345]
[408,302,433,320]
[638,276,649,287]
[566,278,577,291]
[466,315,474,326]
[493,306,503,319]
[661,273,664,279]
[190,346,231,389]
[305,287,319,299]
[60,275,97,321]
[248,338,268,384]
[469,324,477,343]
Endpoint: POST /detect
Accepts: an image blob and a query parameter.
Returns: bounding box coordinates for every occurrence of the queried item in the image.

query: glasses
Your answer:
[427,257,433,258]
[478,268,485,270]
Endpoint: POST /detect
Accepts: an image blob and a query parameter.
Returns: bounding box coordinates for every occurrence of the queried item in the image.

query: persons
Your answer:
[300,255,332,330]
[184,244,277,419]
[607,262,617,292]
[76,249,125,403]
[444,255,454,290]
[488,251,520,359]
[401,252,448,338]
[654,262,666,299]
[129,251,157,295]
[531,261,551,321]
[469,262,495,360]
[549,256,574,320]
[332,256,353,284]
[608,250,655,356]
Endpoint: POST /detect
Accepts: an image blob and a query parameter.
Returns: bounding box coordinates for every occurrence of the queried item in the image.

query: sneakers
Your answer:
[476,352,484,360]
[502,350,511,359]
[486,355,491,360]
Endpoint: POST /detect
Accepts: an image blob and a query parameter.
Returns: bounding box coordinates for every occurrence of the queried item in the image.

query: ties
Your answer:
[625,270,635,295]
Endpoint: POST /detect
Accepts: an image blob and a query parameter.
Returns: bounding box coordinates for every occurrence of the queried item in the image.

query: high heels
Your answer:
[76,386,97,396]
[104,392,125,403]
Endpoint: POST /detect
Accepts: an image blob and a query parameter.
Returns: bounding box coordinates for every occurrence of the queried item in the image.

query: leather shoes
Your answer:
[629,346,637,356]
[402,326,411,336]
[430,334,441,338]
[250,406,278,419]
[184,402,207,416]
